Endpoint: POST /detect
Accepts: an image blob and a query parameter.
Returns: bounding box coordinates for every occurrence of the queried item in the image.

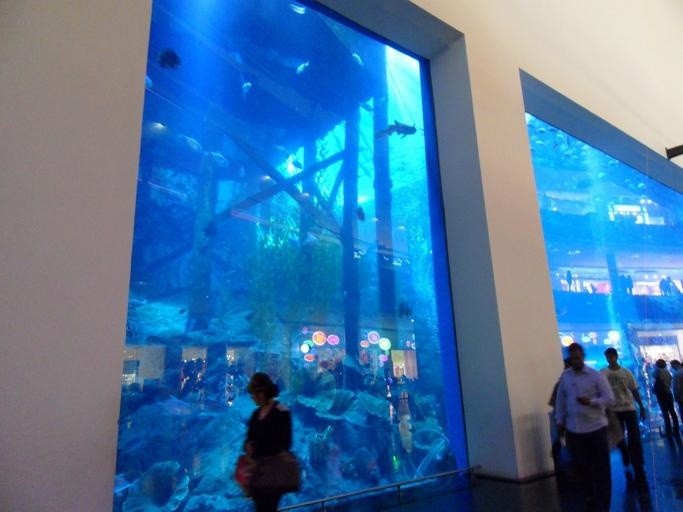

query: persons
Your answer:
[547,342,613,511]
[670,359,682,424]
[658,275,676,296]
[651,358,680,437]
[619,273,634,295]
[564,269,597,295]
[239,372,292,511]
[548,356,572,458]
[597,347,646,472]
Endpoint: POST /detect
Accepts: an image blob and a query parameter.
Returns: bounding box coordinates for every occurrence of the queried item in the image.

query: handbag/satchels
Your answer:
[236,452,301,492]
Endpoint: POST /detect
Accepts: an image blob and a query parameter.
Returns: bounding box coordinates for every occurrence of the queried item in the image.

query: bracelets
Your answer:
[640,406,645,412]
